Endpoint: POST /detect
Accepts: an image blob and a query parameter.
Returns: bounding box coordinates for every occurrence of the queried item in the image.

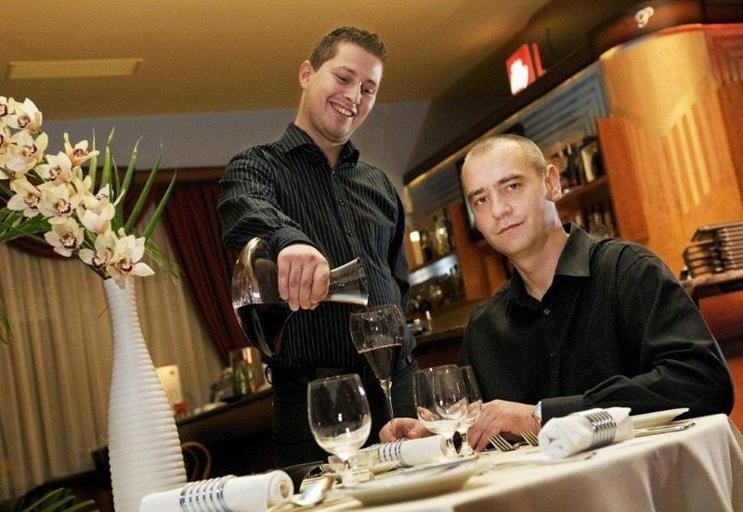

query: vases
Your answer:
[102,275,187,512]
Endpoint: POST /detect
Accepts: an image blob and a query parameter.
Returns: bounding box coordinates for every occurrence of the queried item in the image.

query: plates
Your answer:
[337,458,477,506]
[630,406,690,428]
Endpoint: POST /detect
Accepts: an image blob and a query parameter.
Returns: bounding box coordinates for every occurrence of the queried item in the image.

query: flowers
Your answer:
[0,95,182,290]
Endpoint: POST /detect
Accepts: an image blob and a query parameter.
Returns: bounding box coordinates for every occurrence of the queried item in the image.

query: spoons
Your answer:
[266,474,334,511]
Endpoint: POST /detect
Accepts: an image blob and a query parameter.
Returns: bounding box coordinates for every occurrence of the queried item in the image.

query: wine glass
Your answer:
[349,302,409,444]
[412,363,485,457]
[306,372,375,480]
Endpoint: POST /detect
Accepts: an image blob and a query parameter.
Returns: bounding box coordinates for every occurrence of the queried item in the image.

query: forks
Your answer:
[521,430,539,447]
[488,433,527,452]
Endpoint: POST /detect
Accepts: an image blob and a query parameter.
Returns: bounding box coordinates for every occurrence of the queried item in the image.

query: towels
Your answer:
[537,407,633,460]
[368,435,447,466]
[141,470,295,512]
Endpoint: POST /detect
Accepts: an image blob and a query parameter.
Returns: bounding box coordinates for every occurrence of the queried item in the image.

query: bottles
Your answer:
[228,233,370,357]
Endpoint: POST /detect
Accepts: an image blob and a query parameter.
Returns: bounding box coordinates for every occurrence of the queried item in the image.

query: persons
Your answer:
[377,132,737,455]
[215,25,418,469]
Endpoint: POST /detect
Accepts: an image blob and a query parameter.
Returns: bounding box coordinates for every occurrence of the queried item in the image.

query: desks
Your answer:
[92,385,274,503]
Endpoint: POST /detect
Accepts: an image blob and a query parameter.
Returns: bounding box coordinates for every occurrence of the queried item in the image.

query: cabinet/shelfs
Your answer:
[403,192,509,316]
[543,118,647,241]
